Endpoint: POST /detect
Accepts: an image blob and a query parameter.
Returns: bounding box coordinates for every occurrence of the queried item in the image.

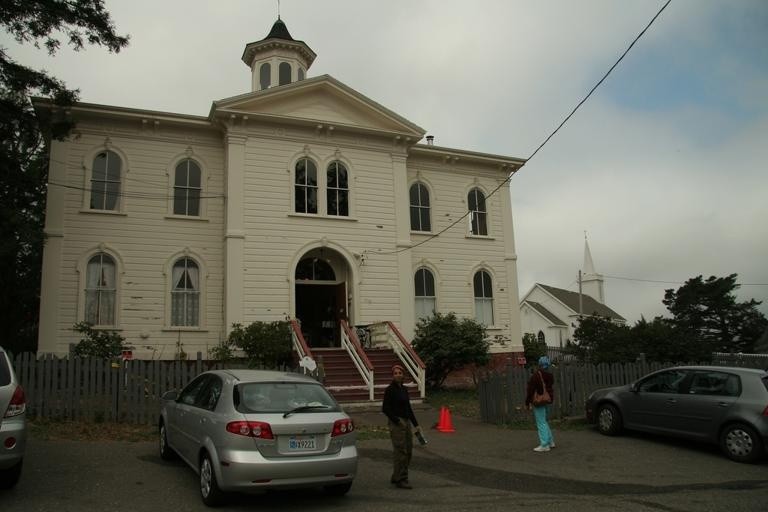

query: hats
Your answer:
[158,369,360,506]
[1,344,27,484]
[584,364,767,463]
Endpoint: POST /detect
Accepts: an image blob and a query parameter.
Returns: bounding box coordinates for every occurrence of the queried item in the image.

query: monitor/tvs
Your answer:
[415,432,428,445]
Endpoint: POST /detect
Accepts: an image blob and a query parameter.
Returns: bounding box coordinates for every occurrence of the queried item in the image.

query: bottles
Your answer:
[539,357,550,369]
[392,365,404,373]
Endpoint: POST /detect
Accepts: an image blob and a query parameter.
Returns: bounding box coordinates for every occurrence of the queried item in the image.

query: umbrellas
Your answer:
[391,480,411,489]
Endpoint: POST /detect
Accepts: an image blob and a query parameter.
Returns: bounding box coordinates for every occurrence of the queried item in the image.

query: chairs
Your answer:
[534,443,555,451]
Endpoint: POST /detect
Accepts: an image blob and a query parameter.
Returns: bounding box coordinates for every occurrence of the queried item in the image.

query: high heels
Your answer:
[436,405,455,433]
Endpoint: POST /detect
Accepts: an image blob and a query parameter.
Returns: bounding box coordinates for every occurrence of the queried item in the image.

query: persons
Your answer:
[383,365,420,490]
[539,354,551,370]
[526,361,556,451]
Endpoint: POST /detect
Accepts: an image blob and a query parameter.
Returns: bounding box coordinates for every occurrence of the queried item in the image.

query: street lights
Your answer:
[534,392,551,406]
